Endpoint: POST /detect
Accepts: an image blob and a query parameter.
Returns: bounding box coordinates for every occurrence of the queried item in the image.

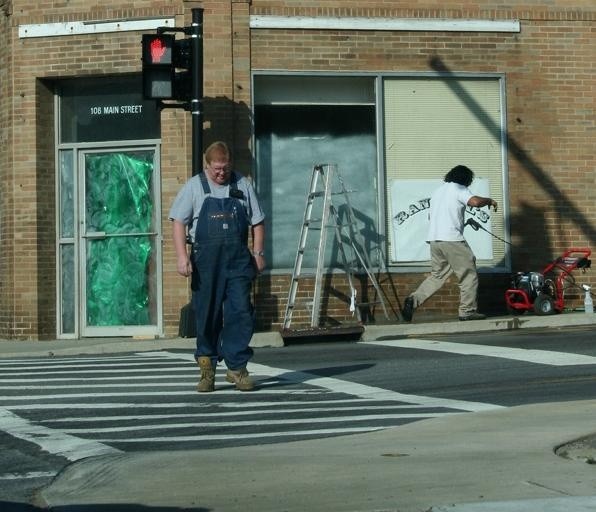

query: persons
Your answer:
[401,164,499,323]
[167,141,267,393]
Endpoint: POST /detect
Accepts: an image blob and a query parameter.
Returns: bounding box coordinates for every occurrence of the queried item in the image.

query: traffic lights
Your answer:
[146,29,178,105]
[168,38,192,105]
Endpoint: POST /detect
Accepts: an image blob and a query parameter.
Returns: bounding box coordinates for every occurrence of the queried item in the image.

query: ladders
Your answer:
[283,160,392,328]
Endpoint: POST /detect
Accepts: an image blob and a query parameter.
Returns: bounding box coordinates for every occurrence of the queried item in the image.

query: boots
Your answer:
[226,366,253,390]
[194,356,216,391]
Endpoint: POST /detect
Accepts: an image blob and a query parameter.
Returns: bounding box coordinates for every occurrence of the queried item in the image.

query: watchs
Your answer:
[252,250,265,258]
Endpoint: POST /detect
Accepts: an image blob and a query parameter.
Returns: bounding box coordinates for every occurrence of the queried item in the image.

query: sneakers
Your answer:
[402,297,413,321]
[459,312,486,320]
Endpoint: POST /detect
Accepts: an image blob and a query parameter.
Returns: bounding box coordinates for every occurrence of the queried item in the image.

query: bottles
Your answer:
[584,290,594,314]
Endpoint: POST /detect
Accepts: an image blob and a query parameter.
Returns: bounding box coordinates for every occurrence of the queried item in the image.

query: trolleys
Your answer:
[504,249,591,316]
